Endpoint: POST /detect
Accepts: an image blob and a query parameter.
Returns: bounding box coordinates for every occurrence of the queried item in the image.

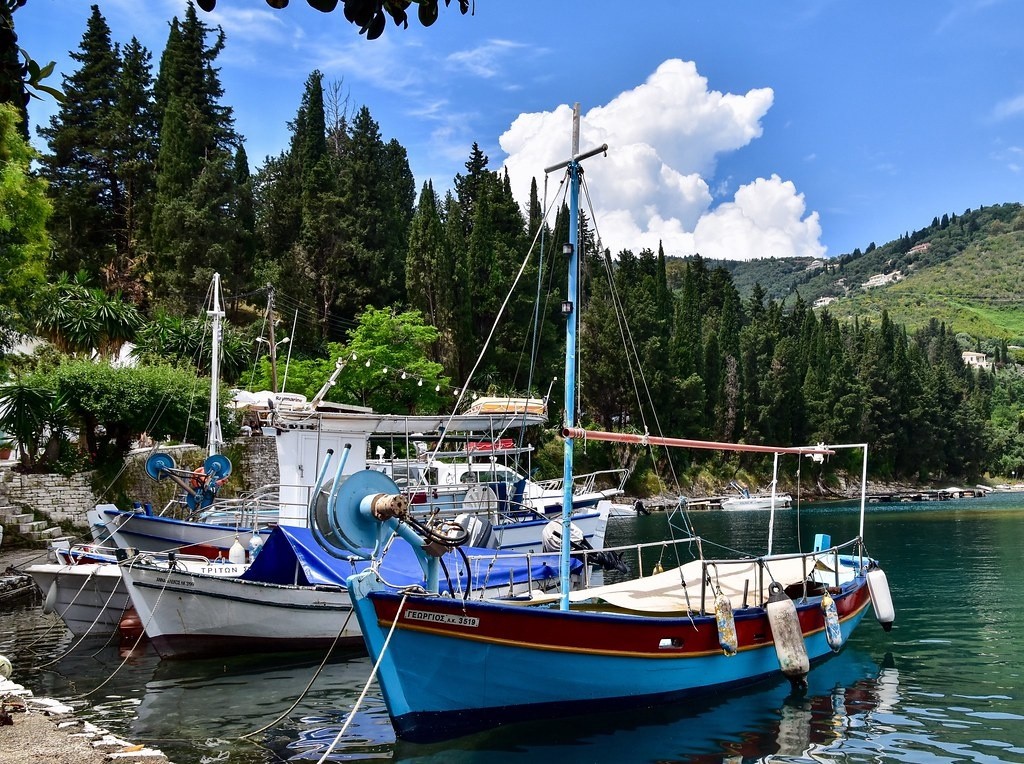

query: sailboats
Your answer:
[21,271,628,660]
[309,101,898,745]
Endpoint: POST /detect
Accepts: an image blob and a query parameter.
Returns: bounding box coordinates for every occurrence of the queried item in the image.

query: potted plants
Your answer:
[0,442,16,460]
